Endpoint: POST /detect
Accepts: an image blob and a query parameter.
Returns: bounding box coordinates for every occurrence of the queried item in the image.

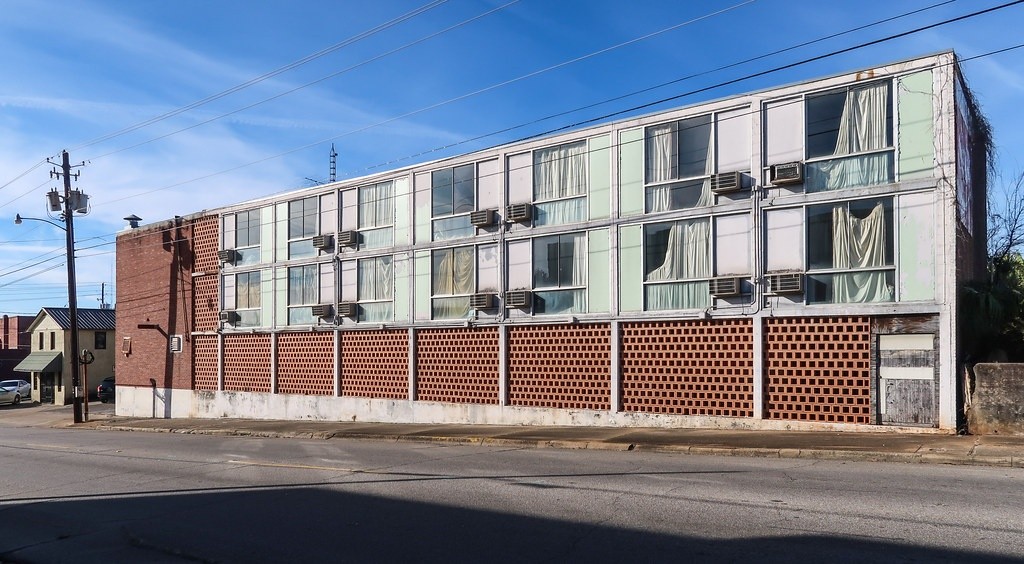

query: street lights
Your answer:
[14,213,83,423]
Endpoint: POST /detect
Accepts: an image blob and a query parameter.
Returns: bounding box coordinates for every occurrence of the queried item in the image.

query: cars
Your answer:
[0,385,21,408]
[0,380,31,400]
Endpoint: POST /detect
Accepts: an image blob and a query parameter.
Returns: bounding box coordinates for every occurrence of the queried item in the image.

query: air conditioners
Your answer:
[217,250,235,263]
[708,277,740,297]
[311,305,332,318]
[338,230,357,246]
[506,204,531,220]
[219,311,235,323]
[338,302,356,317]
[769,274,804,294]
[504,290,530,307]
[312,235,334,249]
[711,171,742,192]
[469,294,493,308]
[470,208,494,225]
[769,160,802,184]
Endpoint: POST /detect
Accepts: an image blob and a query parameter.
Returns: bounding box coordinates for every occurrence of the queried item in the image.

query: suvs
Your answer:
[96,376,115,403]
